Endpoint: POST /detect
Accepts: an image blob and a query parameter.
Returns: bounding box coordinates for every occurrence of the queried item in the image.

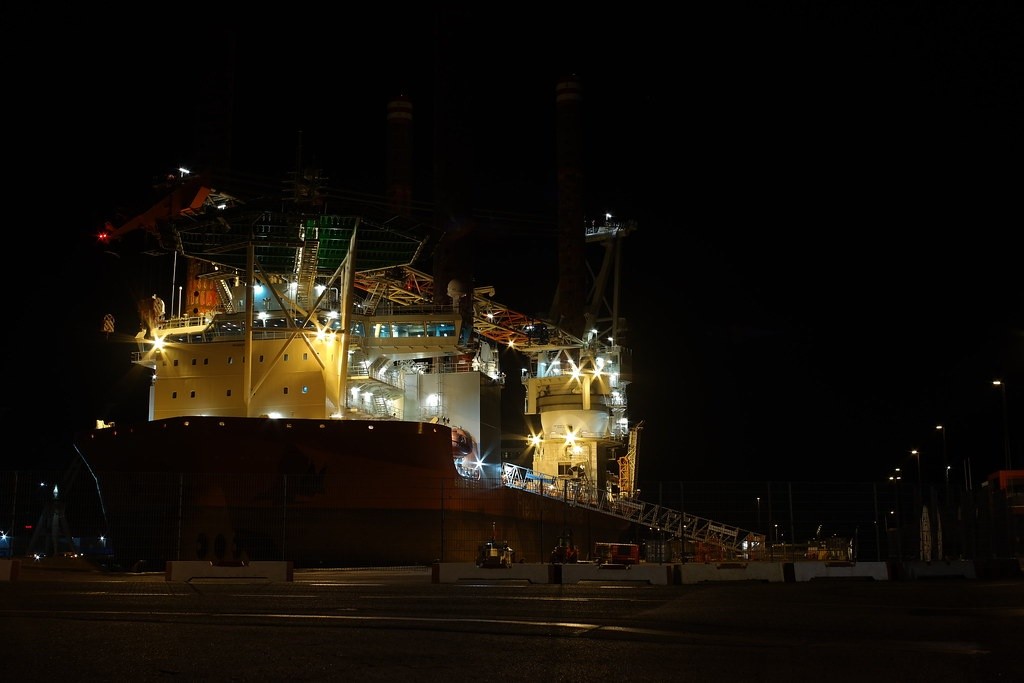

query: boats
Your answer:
[83,413,765,562]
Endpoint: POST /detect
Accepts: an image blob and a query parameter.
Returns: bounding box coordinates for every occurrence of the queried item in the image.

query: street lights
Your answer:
[889,476,901,483]
[993,380,1011,471]
[912,450,920,481]
[936,425,946,451]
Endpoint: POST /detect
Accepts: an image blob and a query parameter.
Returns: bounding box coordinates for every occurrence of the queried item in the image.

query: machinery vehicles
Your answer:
[550,528,577,564]
